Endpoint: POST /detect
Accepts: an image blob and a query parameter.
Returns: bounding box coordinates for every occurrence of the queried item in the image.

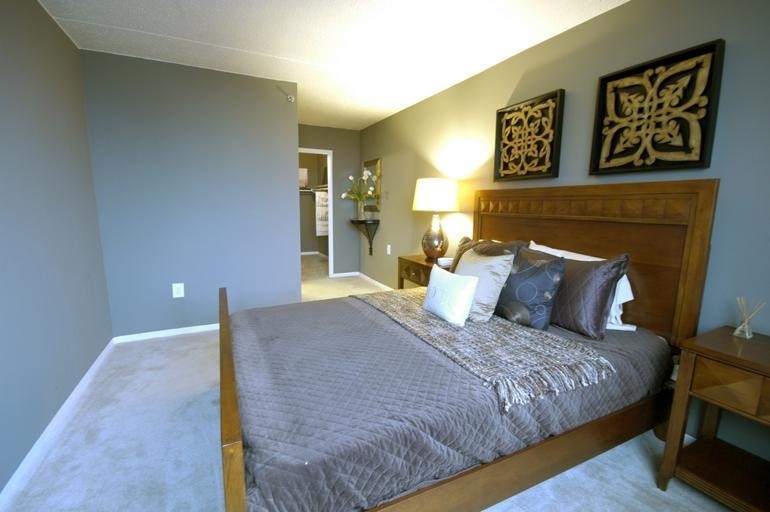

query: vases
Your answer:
[358,200,367,220]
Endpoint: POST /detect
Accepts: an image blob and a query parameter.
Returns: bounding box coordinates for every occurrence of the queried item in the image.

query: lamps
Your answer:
[411,177,460,262]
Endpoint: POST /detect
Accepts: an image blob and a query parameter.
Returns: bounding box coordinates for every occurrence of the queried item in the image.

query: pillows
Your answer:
[517,245,631,343]
[449,235,531,274]
[492,255,568,332]
[453,248,515,324]
[528,238,639,333]
[422,264,481,328]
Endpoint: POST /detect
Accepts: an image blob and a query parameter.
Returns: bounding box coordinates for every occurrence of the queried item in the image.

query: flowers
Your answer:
[339,167,382,203]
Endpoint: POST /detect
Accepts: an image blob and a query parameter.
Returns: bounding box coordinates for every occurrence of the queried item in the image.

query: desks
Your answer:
[397,254,451,289]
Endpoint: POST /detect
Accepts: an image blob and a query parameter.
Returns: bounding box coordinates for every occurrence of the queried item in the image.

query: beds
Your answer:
[215,177,720,511]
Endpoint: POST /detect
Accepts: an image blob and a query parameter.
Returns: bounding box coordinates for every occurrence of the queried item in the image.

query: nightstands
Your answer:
[655,322,770,511]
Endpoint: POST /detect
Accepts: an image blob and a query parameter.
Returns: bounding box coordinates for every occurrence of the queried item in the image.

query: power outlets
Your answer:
[387,244,392,256]
[171,281,186,299]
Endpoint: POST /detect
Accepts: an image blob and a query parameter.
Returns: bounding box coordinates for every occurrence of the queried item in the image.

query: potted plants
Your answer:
[362,205,382,220]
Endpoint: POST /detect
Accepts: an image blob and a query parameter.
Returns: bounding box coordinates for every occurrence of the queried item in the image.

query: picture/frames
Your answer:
[588,37,726,176]
[493,88,565,183]
[362,157,383,212]
